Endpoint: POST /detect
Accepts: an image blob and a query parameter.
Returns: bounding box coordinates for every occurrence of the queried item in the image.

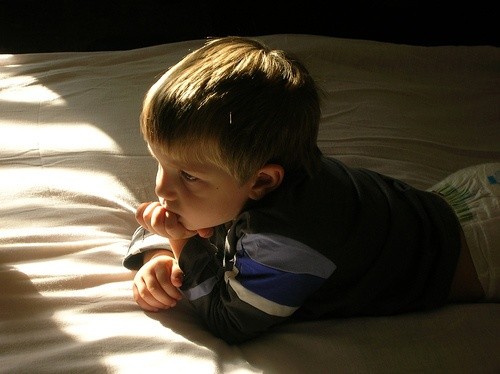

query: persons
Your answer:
[121,34,500,346]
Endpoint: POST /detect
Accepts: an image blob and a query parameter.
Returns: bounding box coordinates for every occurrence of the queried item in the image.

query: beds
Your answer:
[2,35,497,370]
